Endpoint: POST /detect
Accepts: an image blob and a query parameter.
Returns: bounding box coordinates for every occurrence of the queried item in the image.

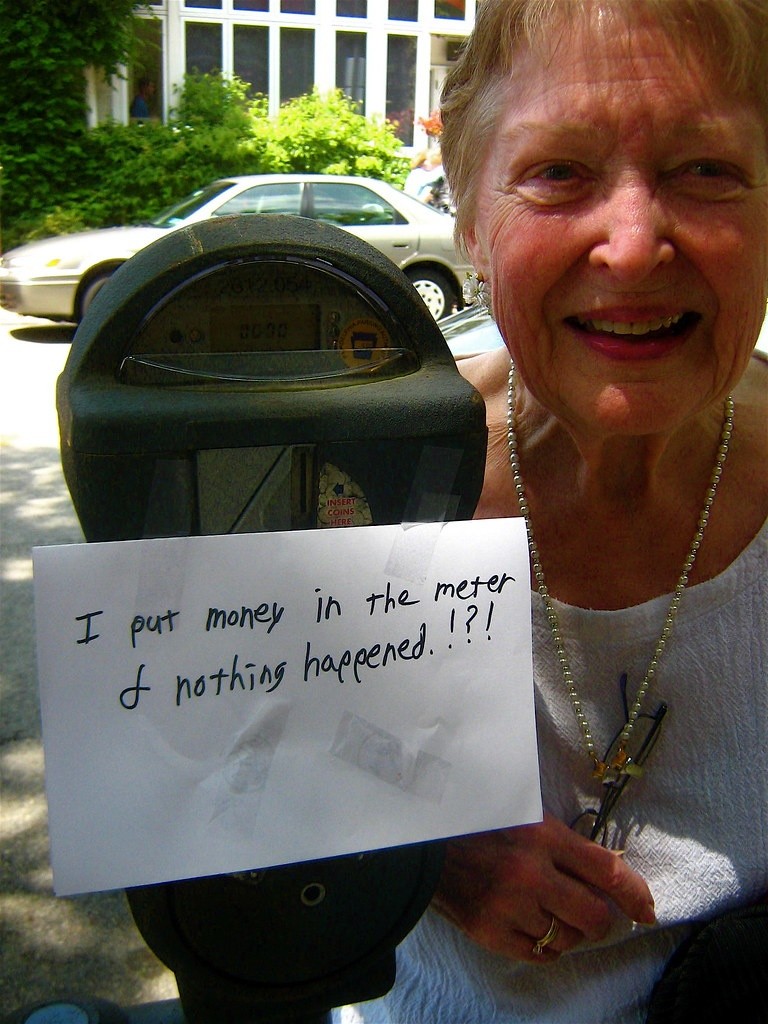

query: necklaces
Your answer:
[507,358,734,784]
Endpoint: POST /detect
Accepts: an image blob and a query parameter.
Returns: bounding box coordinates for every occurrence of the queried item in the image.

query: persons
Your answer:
[403,146,457,218]
[330,0,768,1024]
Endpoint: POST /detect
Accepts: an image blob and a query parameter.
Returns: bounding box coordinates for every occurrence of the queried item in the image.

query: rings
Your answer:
[533,917,560,956]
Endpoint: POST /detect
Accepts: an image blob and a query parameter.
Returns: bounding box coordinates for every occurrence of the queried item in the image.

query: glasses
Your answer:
[569,672,669,848]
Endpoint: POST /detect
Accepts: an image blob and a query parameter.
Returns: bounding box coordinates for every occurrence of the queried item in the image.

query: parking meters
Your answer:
[58,213,487,1003]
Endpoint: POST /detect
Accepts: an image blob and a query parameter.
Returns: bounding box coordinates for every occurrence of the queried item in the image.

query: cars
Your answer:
[1,174,481,328]
